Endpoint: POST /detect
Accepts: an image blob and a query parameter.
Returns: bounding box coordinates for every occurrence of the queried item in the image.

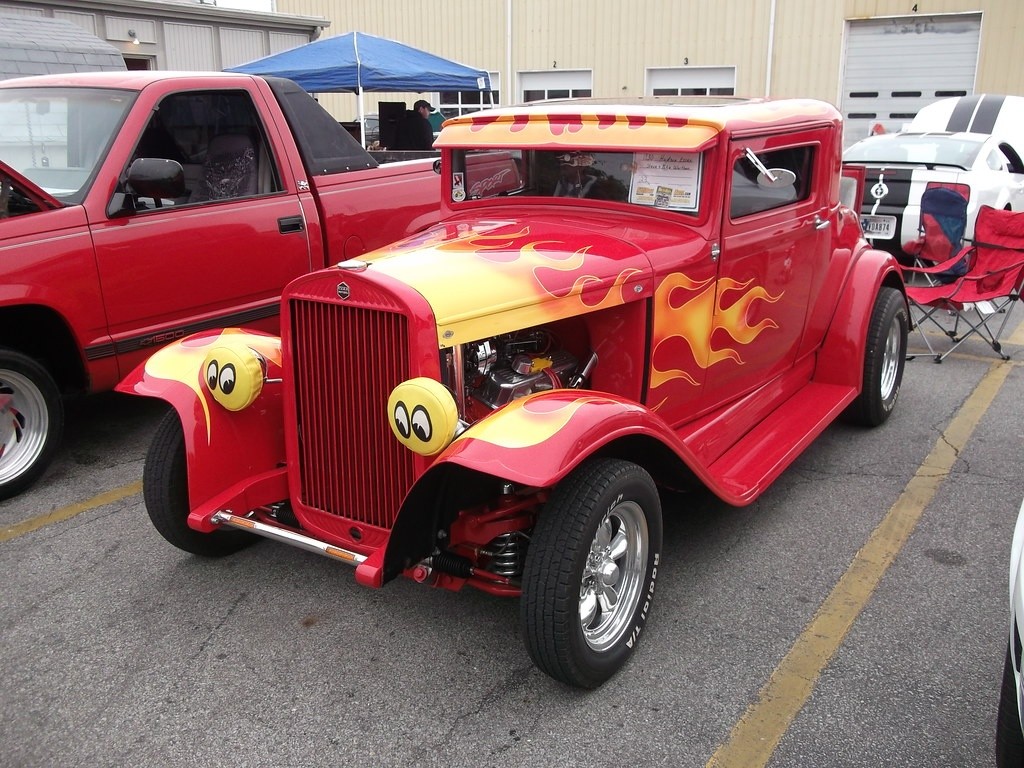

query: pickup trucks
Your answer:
[0,69,523,505]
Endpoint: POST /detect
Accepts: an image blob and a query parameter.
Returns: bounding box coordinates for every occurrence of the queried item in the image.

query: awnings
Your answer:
[0,14,130,97]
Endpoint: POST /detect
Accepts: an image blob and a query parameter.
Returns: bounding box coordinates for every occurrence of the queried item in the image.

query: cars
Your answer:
[113,95,913,693]
[841,95,1024,263]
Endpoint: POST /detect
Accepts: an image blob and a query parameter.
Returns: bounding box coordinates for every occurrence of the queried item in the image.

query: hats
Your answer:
[414,100,436,111]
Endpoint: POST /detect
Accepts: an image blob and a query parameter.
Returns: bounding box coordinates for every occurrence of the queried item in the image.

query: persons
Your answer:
[393,100,436,160]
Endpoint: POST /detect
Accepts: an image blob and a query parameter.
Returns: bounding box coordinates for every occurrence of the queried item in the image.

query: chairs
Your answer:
[185,133,260,204]
[902,180,971,288]
[551,158,610,202]
[901,204,1024,364]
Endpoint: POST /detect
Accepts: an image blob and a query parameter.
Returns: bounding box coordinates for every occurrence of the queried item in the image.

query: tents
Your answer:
[221,31,495,149]
